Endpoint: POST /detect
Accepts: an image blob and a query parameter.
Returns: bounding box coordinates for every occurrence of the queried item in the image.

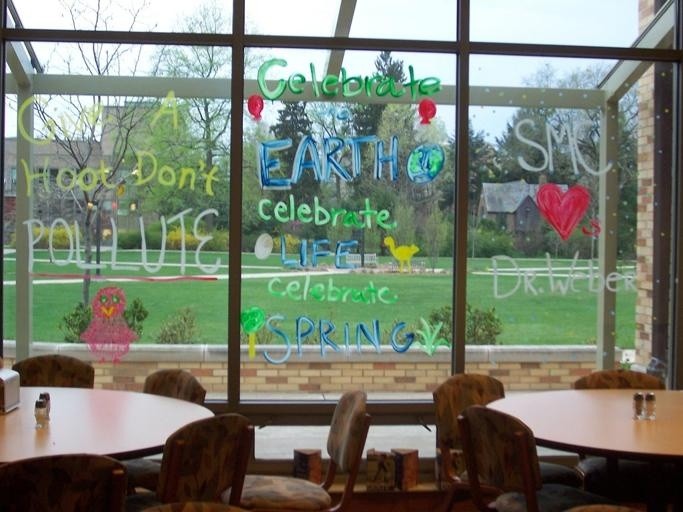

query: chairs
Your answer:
[225,390,371,510]
[435,372,585,510]
[124,368,206,492]
[1,452,130,511]
[9,356,96,388]
[129,414,256,505]
[574,368,662,494]
[456,404,642,512]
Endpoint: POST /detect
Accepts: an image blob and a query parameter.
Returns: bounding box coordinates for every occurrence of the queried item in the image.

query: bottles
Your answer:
[633,393,646,418]
[34,399,46,425]
[645,392,656,420]
[38,393,50,417]
